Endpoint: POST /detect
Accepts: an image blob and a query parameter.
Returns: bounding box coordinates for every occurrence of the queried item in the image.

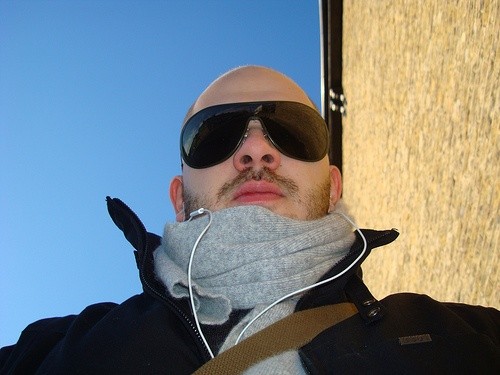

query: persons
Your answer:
[0,62,500,375]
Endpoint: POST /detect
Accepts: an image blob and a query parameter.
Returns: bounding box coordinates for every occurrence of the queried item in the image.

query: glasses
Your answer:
[180,101,329,169]
